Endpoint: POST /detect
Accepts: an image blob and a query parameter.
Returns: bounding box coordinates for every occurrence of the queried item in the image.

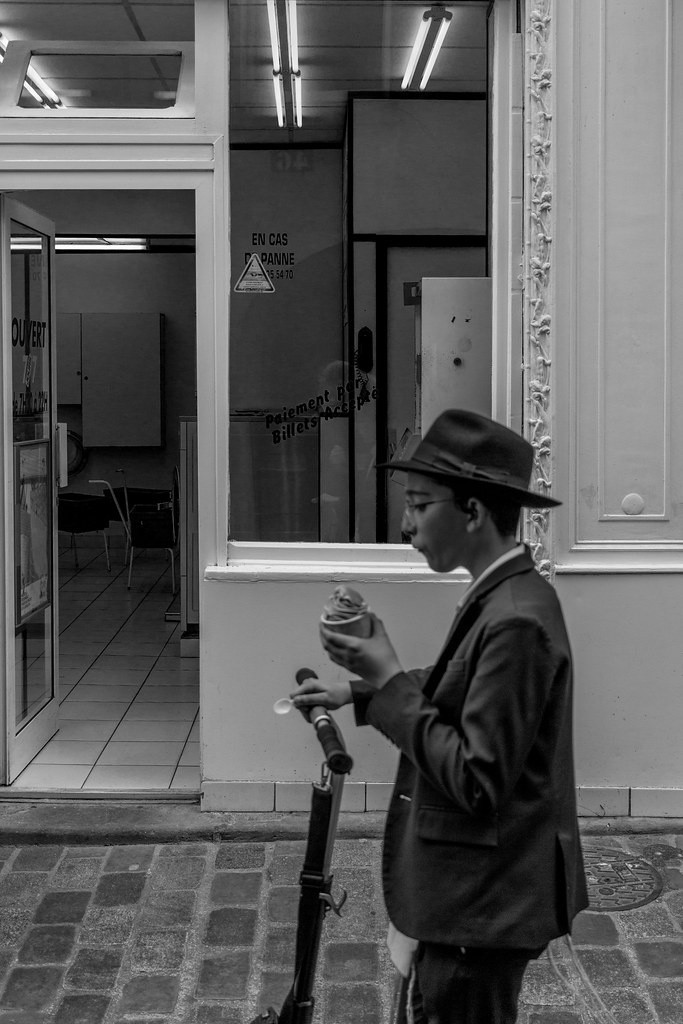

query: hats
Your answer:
[371,409,562,508]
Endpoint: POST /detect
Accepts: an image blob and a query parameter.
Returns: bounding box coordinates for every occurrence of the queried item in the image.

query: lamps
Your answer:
[400,5,453,91]
[0,27,62,109]
[264,0,302,129]
[9,237,150,250]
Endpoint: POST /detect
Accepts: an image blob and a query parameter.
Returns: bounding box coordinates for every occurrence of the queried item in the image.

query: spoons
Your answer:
[273,696,294,716]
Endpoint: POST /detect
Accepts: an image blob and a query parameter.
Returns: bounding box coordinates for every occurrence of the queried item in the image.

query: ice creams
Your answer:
[320,584,372,639]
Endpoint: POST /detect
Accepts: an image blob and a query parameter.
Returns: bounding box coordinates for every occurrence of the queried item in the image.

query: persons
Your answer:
[289,408,589,1024]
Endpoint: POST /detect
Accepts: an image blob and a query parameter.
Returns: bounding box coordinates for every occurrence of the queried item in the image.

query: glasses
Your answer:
[402,496,462,521]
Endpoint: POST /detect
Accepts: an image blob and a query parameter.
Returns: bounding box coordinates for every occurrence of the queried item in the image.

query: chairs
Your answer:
[87,468,177,595]
[57,492,111,571]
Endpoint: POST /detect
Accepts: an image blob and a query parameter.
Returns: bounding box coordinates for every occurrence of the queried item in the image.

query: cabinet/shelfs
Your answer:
[56,312,163,450]
[402,276,491,437]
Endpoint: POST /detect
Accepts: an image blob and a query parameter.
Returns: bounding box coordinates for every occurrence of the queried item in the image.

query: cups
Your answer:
[322,604,373,640]
